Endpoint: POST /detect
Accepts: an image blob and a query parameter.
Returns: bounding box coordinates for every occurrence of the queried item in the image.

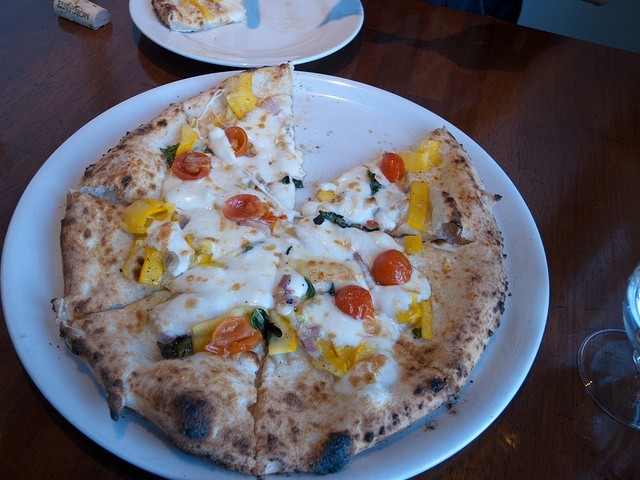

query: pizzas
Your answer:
[151,0,247,33]
[52,65,508,477]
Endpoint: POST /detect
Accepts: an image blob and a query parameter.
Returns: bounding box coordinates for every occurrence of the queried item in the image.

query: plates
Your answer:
[129,0,364,66]
[0,68,549,475]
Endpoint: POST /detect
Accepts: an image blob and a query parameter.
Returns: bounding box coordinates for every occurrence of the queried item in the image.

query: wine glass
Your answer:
[579,267,640,433]
